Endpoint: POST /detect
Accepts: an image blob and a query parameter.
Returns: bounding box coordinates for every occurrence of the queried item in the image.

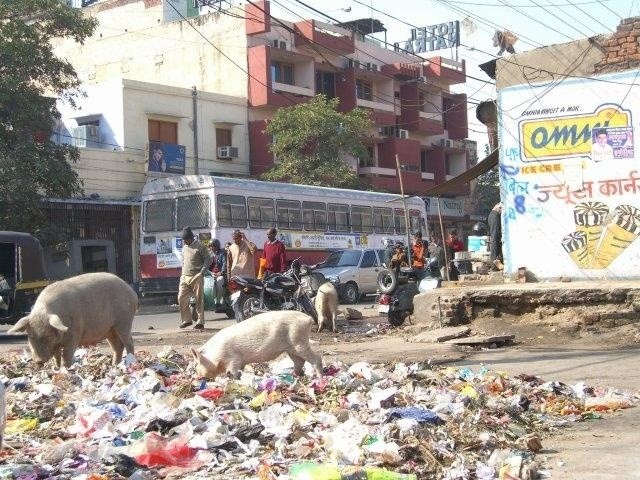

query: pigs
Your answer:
[315,282,338,333]
[190,310,323,380]
[7,272,138,368]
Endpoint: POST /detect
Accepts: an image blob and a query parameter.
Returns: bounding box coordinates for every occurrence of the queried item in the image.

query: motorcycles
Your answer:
[226,255,318,326]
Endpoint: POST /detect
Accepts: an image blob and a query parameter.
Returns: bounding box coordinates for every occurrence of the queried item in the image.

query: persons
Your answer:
[383,228,465,269]
[488,202,502,257]
[149,146,167,171]
[209,227,287,307]
[593,129,614,161]
[178,226,212,330]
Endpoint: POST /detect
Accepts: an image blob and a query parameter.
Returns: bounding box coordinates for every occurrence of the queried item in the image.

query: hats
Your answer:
[181,226,194,239]
[208,239,220,248]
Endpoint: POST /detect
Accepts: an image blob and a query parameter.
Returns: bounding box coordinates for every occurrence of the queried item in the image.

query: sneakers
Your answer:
[178,323,204,330]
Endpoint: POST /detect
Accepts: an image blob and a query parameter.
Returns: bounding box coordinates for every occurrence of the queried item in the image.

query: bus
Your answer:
[135,172,430,305]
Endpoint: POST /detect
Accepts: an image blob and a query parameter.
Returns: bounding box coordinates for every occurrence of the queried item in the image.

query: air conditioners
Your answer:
[395,129,409,139]
[440,138,454,148]
[219,146,239,159]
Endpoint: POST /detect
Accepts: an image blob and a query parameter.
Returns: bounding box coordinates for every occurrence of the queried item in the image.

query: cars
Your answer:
[310,247,387,305]
